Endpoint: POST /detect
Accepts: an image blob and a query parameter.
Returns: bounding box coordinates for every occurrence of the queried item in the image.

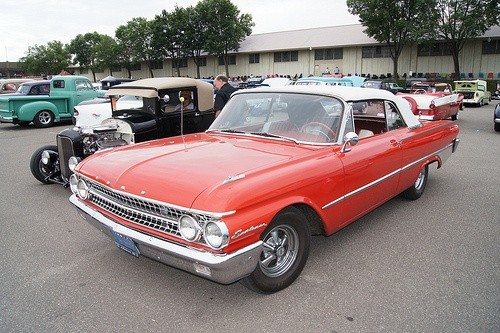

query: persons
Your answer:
[250,74,253,78]
[174,90,194,111]
[333,66,339,75]
[326,67,329,74]
[213,74,235,117]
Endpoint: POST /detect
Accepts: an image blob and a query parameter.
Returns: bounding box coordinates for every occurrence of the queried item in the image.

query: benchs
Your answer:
[311,116,386,138]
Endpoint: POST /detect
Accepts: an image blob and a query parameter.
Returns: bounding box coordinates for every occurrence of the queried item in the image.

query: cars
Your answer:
[453,80,492,106]
[69,76,460,294]
[30,77,216,189]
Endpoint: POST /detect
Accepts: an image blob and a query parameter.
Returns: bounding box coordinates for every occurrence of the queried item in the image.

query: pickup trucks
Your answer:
[0,75,120,128]
[396,81,464,120]
